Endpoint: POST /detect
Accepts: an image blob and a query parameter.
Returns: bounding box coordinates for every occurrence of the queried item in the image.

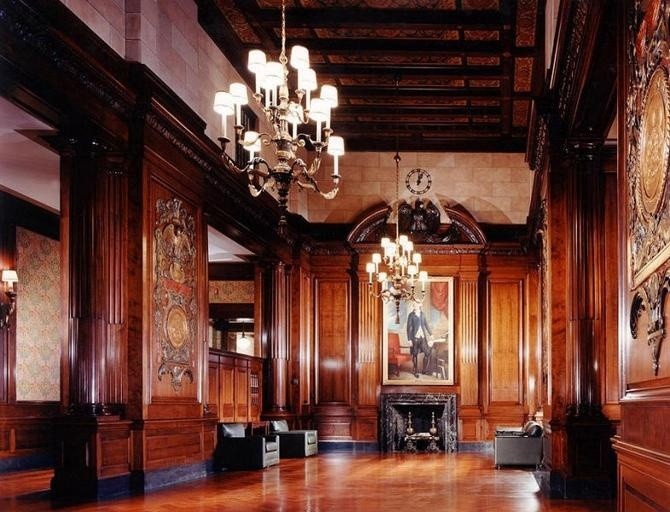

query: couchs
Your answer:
[493,420,547,473]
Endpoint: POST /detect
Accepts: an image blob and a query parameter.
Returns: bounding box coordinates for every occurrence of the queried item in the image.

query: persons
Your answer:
[406,300,434,376]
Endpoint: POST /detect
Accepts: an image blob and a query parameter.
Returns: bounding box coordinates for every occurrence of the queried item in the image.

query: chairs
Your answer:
[217,422,280,469]
[266,418,319,457]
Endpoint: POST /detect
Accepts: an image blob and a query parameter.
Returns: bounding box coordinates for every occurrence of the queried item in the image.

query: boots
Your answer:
[412,357,420,379]
[422,357,430,374]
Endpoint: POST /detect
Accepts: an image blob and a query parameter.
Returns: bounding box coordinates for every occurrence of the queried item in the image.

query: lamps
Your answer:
[0,269,20,331]
[237,320,252,351]
[365,74,429,329]
[209,0,347,245]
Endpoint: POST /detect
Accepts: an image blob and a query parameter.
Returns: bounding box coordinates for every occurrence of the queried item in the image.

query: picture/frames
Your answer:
[373,270,461,388]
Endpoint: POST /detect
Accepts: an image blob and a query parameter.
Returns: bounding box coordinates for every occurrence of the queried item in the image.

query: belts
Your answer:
[414,338,426,341]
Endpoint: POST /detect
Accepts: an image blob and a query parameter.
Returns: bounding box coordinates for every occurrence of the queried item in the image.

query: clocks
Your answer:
[405,168,432,195]
